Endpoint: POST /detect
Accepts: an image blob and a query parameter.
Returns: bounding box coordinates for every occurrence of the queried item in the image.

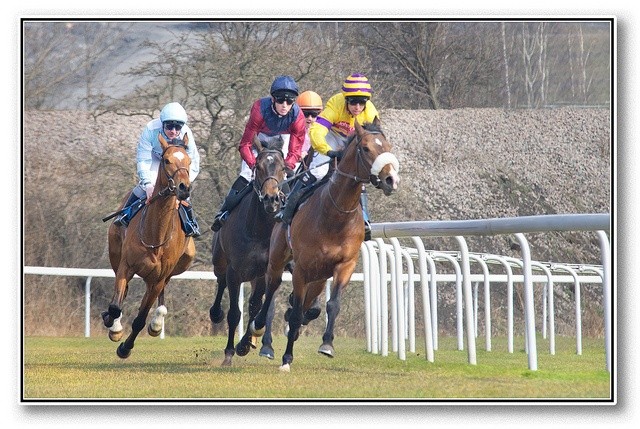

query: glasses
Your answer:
[302,110,321,118]
[348,97,367,105]
[164,123,183,131]
[273,96,295,105]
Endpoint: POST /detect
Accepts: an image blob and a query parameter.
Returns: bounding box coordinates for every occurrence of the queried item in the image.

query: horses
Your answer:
[101,132,195,358]
[210,131,285,368]
[250,114,400,374]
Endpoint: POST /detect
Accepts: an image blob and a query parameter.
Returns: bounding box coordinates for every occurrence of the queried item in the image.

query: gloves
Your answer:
[284,167,294,178]
[327,150,342,160]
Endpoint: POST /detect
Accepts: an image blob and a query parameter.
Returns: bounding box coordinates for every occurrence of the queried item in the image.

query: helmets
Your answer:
[160,102,188,127]
[270,76,298,96]
[341,74,372,100]
[295,91,323,109]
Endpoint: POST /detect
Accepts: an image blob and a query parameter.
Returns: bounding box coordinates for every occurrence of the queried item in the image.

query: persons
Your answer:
[209,74,307,232]
[273,73,383,241]
[113,102,201,237]
[293,90,322,152]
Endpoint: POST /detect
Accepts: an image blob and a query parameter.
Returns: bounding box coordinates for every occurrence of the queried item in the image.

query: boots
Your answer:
[274,179,307,223]
[181,199,201,238]
[211,175,249,232]
[113,191,140,227]
[360,190,370,226]
